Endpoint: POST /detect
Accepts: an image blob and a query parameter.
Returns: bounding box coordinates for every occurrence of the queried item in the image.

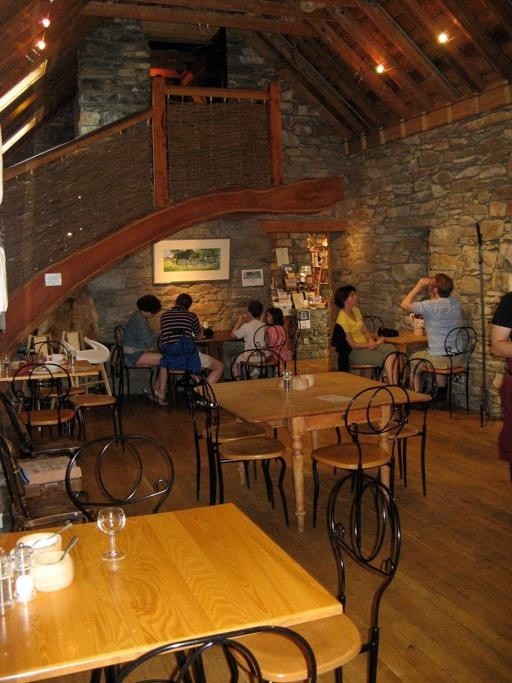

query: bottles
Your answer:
[0,543,37,615]
[3,353,10,373]
[281,370,291,392]
[413,326,423,337]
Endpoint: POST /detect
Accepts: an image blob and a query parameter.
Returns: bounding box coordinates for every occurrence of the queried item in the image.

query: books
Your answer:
[18,455,83,485]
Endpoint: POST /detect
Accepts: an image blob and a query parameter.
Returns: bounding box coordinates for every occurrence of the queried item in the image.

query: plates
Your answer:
[28,367,50,374]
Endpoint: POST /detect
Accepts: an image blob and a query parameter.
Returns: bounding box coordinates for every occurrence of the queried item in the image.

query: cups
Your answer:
[43,353,65,371]
[291,374,314,391]
[18,532,62,557]
[35,550,75,592]
[67,350,77,369]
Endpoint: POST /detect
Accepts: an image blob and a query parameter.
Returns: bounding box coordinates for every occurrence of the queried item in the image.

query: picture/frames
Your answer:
[153,239,230,282]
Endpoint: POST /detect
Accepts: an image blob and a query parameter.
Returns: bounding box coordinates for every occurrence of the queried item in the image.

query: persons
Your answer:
[231,302,270,382]
[401,273,465,410]
[158,293,225,384]
[121,294,169,406]
[489,292,512,463]
[331,285,406,387]
[249,308,292,378]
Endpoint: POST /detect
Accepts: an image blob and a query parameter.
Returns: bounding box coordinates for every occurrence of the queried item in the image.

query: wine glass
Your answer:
[96,508,127,561]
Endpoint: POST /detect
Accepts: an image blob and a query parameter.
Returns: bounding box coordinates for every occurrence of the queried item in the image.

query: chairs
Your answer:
[194,379,289,526]
[421,326,477,417]
[26,342,68,365]
[66,434,175,522]
[0,391,83,459]
[232,349,285,380]
[75,347,125,451]
[226,473,401,683]
[377,352,410,388]
[183,375,271,504]
[12,364,74,438]
[114,326,157,403]
[156,329,195,410]
[311,385,409,544]
[390,359,436,498]
[114,625,317,683]
[284,317,301,374]
[240,325,287,376]
[364,316,383,333]
[0,434,92,532]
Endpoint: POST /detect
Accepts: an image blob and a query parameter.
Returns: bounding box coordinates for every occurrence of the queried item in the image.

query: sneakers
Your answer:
[429,393,453,409]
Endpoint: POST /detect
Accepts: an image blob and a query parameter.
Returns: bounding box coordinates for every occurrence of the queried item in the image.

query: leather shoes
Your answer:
[142,387,154,401]
[150,394,169,406]
